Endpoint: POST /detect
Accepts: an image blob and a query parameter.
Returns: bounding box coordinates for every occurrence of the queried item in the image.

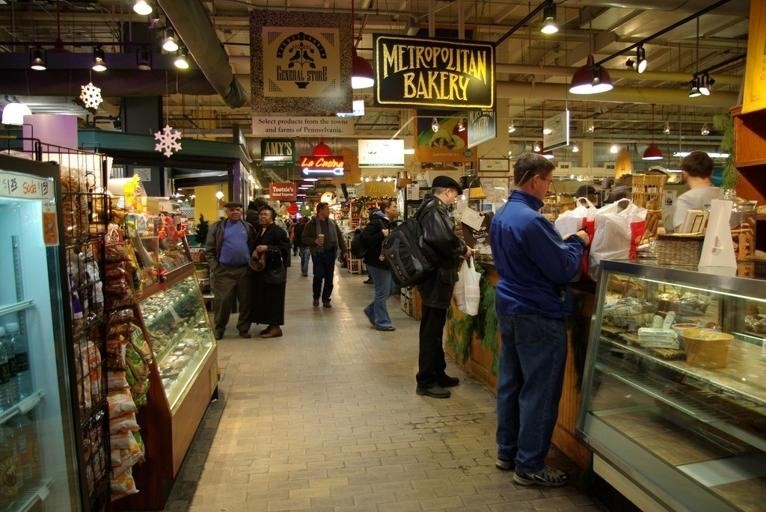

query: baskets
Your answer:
[729,211,757,229]
[655,234,704,267]
[657,292,711,316]
[608,275,643,298]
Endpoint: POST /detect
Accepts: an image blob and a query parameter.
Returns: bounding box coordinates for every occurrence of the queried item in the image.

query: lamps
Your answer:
[135,45,152,72]
[28,44,48,71]
[687,14,714,100]
[537,0,650,98]
[641,103,664,161]
[91,45,107,73]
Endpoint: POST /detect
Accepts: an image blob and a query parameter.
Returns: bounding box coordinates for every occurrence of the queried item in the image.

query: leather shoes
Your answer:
[239,330,251,338]
[437,371,458,387]
[260,325,282,338]
[214,329,224,339]
[416,383,450,398]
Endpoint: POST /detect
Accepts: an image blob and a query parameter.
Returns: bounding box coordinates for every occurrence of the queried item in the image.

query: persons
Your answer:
[355,197,400,330]
[206,202,290,339]
[674,149,732,225]
[290,217,310,277]
[406,174,476,400]
[578,185,597,207]
[490,152,592,487]
[302,202,347,308]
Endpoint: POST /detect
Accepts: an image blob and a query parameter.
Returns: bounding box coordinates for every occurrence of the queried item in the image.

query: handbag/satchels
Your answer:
[248,256,265,271]
[248,245,286,284]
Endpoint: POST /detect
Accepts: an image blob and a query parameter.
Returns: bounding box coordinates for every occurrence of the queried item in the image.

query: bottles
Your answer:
[0,323,43,510]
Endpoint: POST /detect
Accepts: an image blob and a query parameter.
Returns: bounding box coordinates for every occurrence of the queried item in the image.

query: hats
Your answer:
[432,176,462,195]
[224,202,242,208]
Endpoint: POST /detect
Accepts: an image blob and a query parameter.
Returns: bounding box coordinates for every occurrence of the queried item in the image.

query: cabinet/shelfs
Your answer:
[572,257,766,512]
[728,103,766,221]
[136,261,219,483]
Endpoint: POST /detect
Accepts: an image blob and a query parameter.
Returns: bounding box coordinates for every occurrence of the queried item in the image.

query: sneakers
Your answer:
[388,327,395,331]
[512,464,570,486]
[314,298,319,306]
[364,305,374,325]
[364,279,373,283]
[496,457,515,471]
[323,300,331,308]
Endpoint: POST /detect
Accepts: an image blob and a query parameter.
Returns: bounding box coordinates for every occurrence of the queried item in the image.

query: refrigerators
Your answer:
[0,156,87,512]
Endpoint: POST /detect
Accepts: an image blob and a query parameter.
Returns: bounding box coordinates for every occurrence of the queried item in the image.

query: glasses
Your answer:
[528,173,552,186]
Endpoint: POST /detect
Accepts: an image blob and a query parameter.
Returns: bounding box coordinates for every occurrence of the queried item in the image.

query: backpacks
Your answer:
[351,218,384,259]
[379,198,451,287]
